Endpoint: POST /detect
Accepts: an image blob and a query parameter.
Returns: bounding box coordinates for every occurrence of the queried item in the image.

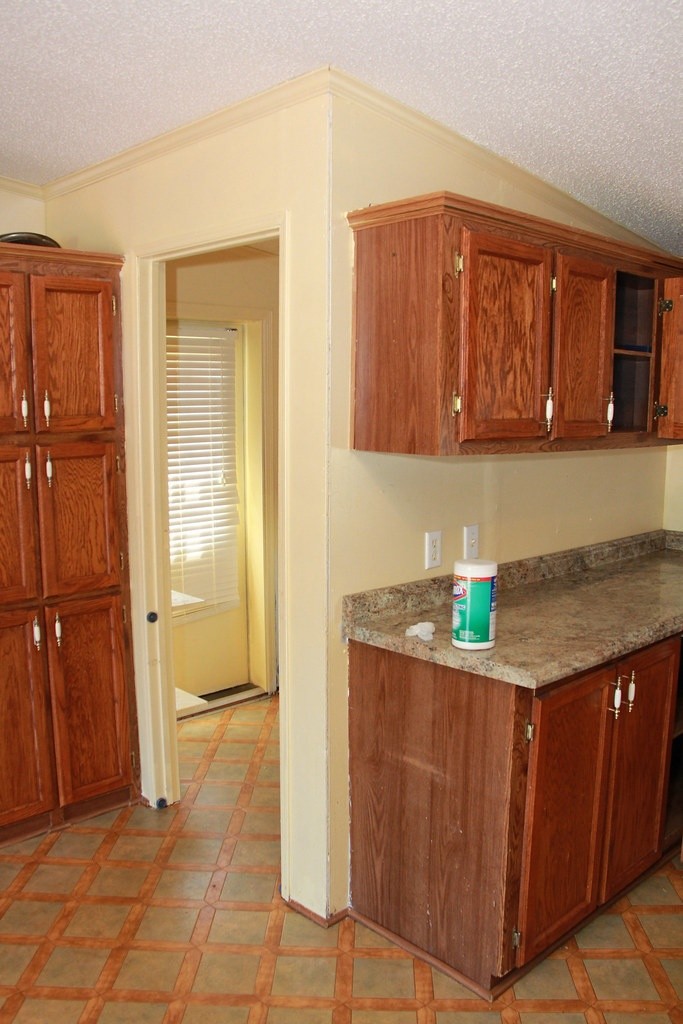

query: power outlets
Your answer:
[463,524,479,561]
[425,530,442,571]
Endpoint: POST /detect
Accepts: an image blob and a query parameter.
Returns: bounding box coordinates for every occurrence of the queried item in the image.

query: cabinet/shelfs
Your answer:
[347,632,682,1005]
[0,231,144,852]
[347,190,683,458]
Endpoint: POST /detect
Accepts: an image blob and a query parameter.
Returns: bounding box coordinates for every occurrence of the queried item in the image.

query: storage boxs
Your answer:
[449,559,500,650]
